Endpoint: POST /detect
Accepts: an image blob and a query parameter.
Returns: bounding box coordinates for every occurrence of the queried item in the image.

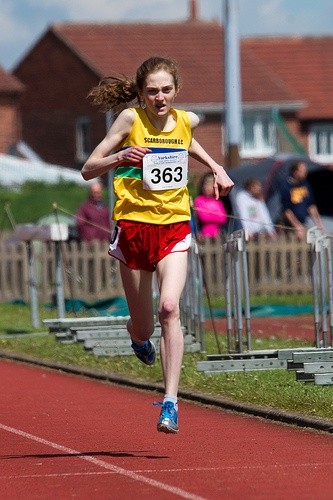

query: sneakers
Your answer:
[153,400,179,434]
[131,338,156,365]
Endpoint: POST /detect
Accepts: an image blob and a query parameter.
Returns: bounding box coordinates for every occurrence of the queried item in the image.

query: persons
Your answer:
[189,162,329,242]
[75,182,114,295]
[80,55,236,435]
